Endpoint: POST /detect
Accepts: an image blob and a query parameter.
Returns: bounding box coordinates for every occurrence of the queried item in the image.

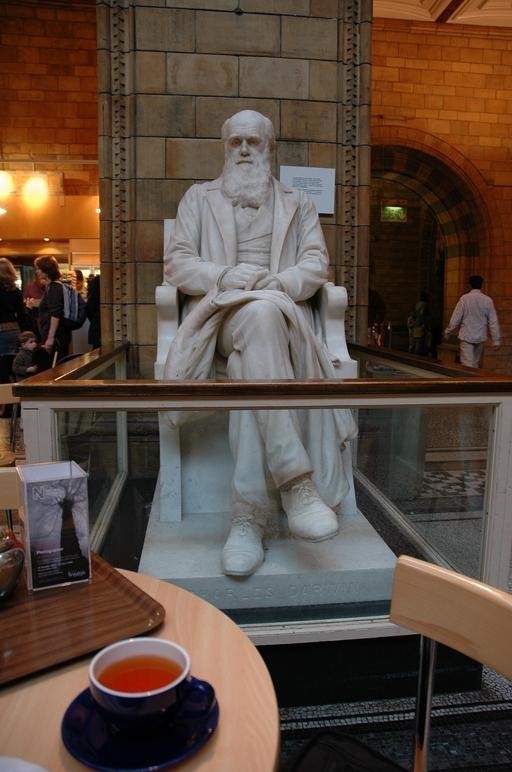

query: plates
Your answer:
[60,676,220,772]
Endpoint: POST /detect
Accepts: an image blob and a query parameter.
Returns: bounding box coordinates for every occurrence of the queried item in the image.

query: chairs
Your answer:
[283,551,511,772]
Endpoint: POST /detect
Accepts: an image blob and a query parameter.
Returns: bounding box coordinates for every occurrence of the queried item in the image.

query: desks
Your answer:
[1,563,294,771]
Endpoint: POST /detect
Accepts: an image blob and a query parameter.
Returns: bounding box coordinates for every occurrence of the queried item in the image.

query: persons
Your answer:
[442,274,504,369]
[365,289,442,362]
[155,106,361,582]
[0,253,103,432]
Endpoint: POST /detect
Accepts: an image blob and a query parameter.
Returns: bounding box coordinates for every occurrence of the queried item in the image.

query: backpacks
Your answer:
[49,280,86,331]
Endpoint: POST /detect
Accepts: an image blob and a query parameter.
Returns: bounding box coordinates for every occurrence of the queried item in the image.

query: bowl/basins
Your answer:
[89,637,192,720]
